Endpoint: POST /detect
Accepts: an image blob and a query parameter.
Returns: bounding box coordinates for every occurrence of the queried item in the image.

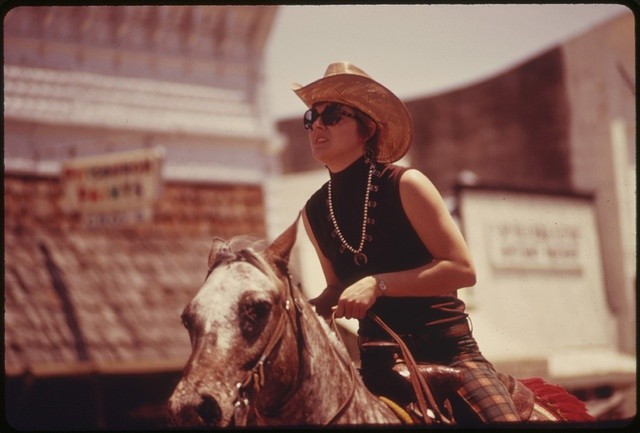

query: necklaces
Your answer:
[327,163,376,254]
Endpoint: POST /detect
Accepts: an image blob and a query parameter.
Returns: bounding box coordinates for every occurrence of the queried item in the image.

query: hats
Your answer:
[291,63,413,165]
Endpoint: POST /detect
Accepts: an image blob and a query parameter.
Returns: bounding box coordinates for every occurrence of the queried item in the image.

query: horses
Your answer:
[168,209,595,424]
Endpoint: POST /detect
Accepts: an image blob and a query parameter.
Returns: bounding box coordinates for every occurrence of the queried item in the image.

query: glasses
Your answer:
[303,104,369,130]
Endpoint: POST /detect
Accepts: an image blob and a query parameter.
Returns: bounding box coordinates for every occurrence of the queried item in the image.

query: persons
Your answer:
[290,62,523,422]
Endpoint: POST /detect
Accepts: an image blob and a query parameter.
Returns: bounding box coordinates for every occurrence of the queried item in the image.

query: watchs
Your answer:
[377,272,386,296]
